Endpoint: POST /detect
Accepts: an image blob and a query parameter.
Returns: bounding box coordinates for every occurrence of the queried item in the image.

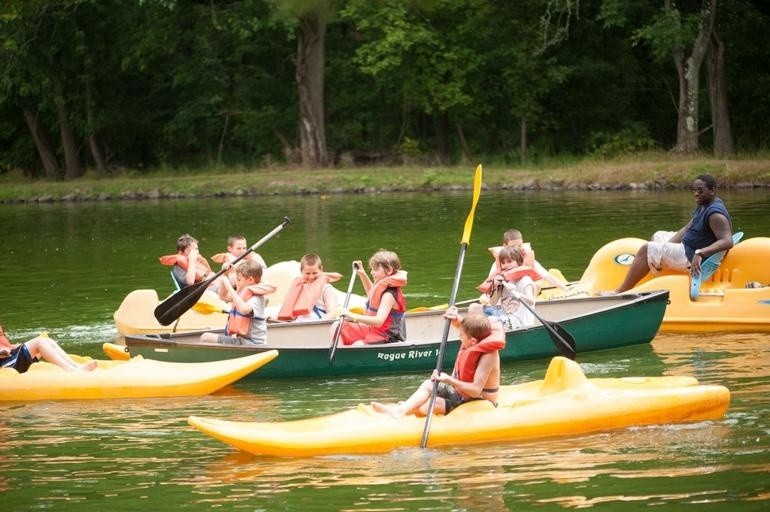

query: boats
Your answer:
[112,262,386,330]
[125,289,690,379]
[558,236,765,332]
[204,355,733,442]
[0,345,291,415]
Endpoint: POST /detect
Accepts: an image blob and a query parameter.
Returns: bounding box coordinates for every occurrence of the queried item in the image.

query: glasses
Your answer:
[692,188,703,194]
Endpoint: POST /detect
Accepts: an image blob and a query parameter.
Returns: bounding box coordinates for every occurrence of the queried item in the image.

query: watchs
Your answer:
[694,246,705,259]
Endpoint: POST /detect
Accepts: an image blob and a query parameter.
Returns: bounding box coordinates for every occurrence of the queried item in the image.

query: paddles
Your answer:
[329,266,357,366]
[502,281,575,360]
[154,216,293,327]
[421,164,482,447]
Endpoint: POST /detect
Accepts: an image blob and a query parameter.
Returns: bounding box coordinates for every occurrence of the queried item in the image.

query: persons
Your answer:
[488,227,571,301]
[329,249,407,347]
[276,252,344,326]
[201,262,275,347]
[595,173,734,298]
[372,314,501,418]
[0,333,99,375]
[212,238,270,300]
[467,246,542,329]
[159,235,230,303]
